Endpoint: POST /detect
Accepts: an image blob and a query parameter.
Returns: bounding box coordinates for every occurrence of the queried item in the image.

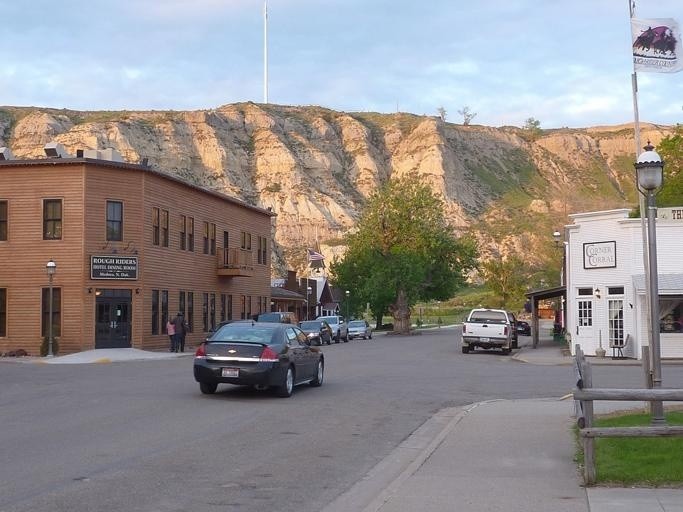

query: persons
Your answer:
[165,319,176,352]
[172,312,189,352]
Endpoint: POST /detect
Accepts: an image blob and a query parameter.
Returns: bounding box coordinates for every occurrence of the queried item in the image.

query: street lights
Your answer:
[552,231,566,330]
[633,140,664,425]
[45,260,55,358]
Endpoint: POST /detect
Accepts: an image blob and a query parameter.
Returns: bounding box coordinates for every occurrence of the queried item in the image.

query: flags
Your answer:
[307,249,326,261]
[629,16,682,74]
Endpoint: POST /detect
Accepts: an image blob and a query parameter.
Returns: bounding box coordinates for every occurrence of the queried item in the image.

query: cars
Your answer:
[517,321,531,336]
[192,312,372,397]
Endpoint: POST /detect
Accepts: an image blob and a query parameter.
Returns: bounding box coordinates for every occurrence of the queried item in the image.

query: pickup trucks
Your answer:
[462,309,517,353]
